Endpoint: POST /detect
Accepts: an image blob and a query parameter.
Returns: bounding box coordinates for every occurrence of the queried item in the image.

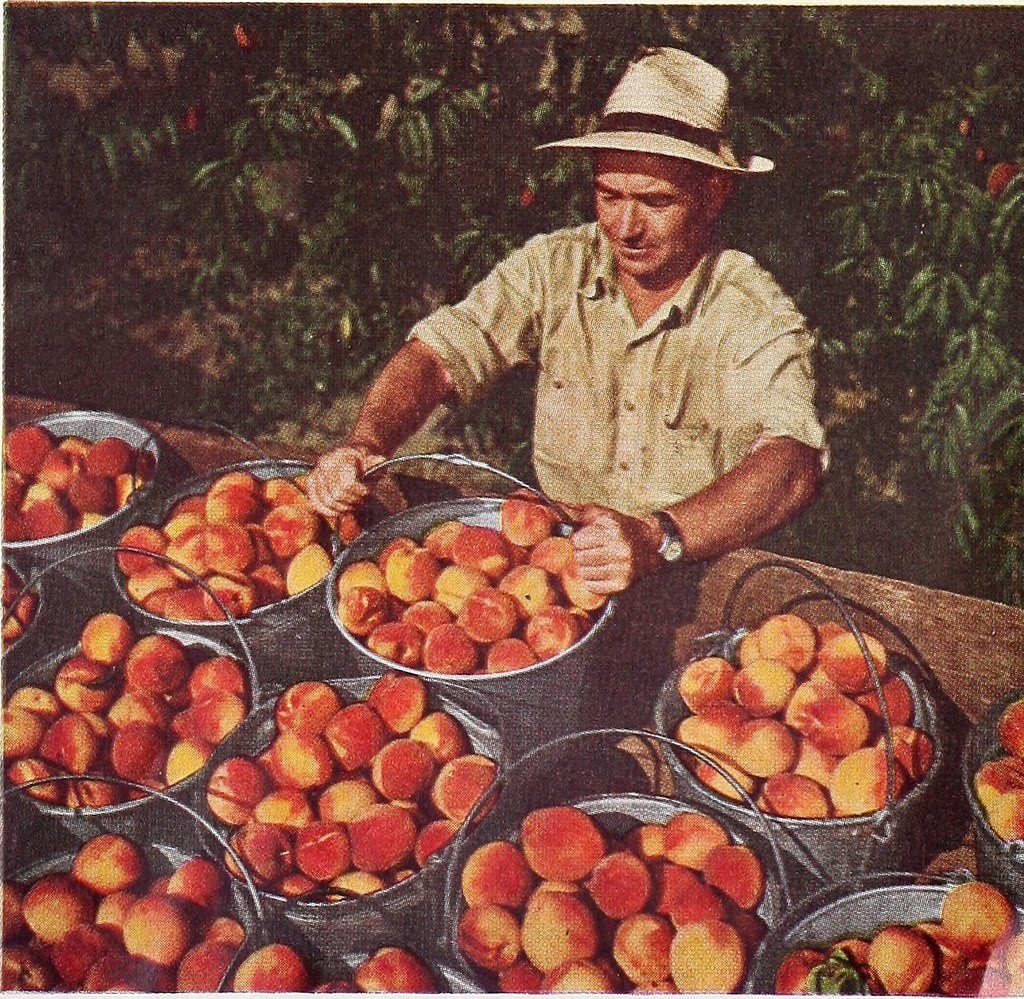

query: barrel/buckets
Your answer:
[3,397,1021,992]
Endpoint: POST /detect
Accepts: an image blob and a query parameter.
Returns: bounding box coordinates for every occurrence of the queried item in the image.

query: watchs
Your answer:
[649,510,685,575]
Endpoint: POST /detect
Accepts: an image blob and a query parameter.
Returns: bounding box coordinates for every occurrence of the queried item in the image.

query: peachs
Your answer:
[2,424,1022,994]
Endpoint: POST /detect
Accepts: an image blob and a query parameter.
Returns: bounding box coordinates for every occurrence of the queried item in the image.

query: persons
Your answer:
[306,46,832,595]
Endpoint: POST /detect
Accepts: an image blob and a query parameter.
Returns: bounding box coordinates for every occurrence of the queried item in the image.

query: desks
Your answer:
[8,393,1023,996]
